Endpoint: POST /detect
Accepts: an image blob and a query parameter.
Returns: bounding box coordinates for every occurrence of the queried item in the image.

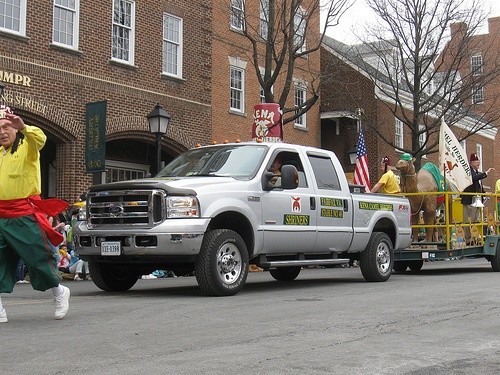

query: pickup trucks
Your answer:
[72,139,411,296]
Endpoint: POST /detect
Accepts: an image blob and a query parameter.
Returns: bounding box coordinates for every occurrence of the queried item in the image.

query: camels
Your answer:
[396,153,453,248]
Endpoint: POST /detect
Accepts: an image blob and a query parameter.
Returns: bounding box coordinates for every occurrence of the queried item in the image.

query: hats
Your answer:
[382,156,391,165]
[470,153,478,160]
[0,104,13,119]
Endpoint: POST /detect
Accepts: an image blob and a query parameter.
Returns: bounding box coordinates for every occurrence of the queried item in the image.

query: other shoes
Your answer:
[86,275,92,280]
[74,276,83,281]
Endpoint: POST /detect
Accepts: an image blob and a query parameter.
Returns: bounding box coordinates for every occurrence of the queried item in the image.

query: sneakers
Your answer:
[0,308,8,323]
[54,285,70,320]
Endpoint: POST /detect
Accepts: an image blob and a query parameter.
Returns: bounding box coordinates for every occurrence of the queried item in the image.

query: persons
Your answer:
[0,103,71,324]
[269,155,288,188]
[461,153,496,246]
[31,203,200,280]
[372,155,404,198]
[415,211,493,242]
[495,178,500,237]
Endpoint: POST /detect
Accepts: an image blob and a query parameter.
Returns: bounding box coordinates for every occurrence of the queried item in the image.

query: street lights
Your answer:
[145,103,171,173]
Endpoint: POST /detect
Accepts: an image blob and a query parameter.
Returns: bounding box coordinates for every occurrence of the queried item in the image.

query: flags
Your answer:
[353,109,371,194]
[437,116,474,199]
